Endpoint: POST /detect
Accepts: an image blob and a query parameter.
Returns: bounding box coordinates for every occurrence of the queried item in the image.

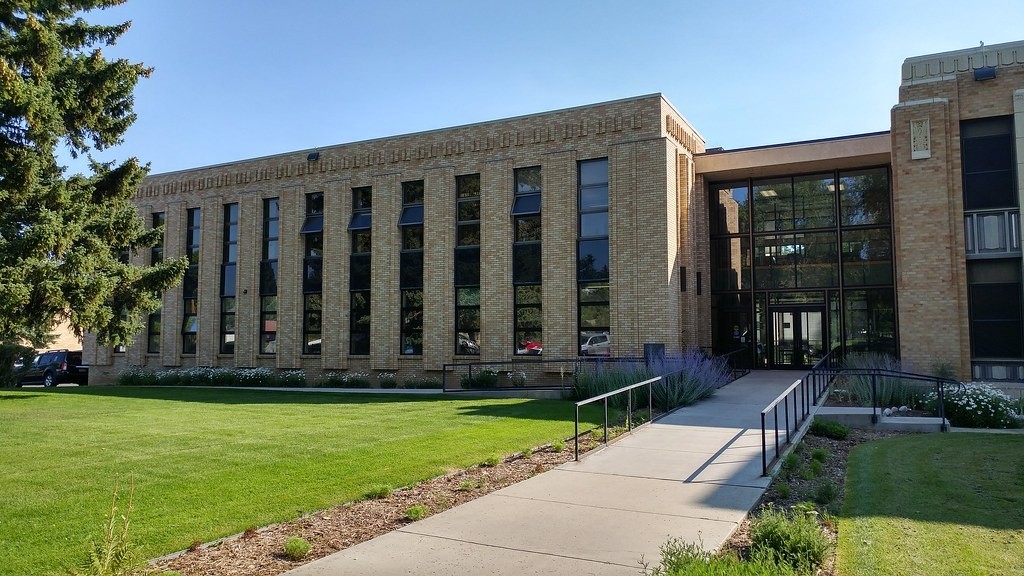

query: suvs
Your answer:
[12,347,87,388]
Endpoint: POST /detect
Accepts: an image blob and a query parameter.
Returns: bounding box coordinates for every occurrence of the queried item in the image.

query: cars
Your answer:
[529,346,542,355]
[459,335,481,351]
[580,334,609,356]
